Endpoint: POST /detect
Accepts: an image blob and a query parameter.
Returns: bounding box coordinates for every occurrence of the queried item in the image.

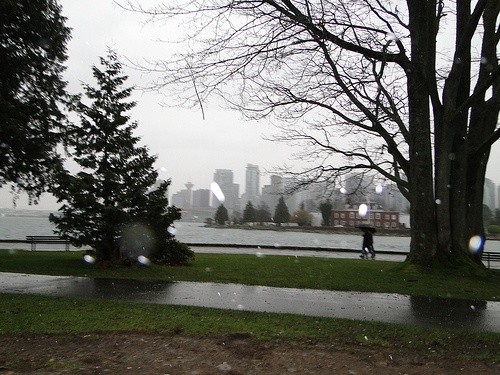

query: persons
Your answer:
[362,229,375,259]
[360,231,375,257]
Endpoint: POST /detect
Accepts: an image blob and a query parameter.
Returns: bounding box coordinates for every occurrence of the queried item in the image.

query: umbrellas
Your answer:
[355,224,377,233]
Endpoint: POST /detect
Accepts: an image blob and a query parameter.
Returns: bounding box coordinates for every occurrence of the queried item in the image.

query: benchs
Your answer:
[482,252,500,268]
[26,236,71,252]
